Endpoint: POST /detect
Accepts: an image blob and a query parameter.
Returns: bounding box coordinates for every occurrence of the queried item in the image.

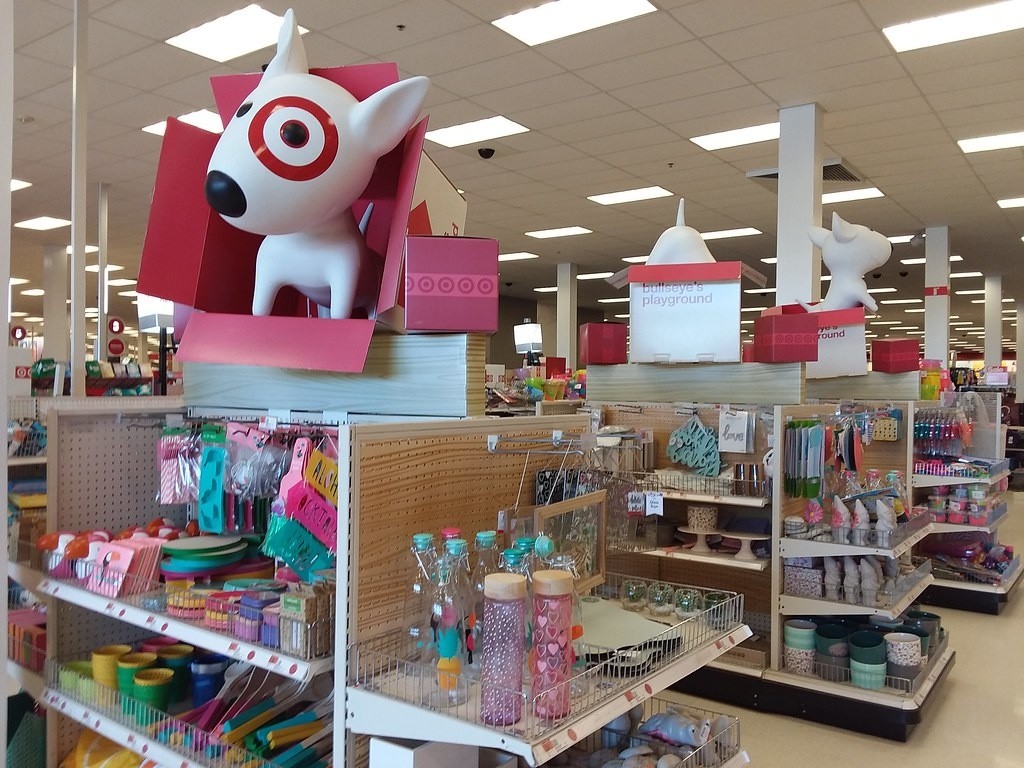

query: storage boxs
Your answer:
[479,746,517,768]
[135,62,921,380]
[368,737,479,768]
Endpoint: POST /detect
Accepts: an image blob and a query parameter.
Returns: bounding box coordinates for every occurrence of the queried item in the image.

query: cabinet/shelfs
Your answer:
[1,363,1024,768]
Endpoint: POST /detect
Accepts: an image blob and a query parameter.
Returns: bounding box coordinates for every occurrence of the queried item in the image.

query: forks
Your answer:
[149,660,333,768]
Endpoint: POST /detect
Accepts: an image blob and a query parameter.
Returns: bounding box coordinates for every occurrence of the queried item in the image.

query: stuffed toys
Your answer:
[584,703,737,768]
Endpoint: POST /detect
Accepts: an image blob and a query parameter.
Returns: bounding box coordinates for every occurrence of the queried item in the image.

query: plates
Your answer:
[167,578,289,629]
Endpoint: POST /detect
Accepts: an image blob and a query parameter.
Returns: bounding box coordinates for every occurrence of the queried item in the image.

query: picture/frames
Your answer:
[502,503,537,548]
[532,487,605,596]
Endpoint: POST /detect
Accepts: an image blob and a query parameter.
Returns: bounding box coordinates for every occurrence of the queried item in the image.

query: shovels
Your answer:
[145,661,334,768]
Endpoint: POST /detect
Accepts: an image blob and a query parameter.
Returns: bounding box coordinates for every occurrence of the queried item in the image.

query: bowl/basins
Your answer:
[784,601,944,689]
[920,384,937,400]
[927,483,989,528]
[60,661,95,699]
[922,359,943,369]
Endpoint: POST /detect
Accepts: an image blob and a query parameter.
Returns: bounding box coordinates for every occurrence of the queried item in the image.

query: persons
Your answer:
[824,496,909,604]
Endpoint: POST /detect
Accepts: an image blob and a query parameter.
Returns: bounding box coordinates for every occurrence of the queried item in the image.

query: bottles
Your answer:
[471,531,498,680]
[838,470,859,498]
[421,557,468,707]
[817,465,835,523]
[888,470,908,511]
[438,528,461,556]
[502,536,541,683]
[399,533,438,676]
[443,539,478,685]
[864,469,885,492]
[535,535,589,697]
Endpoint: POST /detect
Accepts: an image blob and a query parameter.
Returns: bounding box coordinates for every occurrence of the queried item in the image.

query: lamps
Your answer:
[514,318,542,366]
[136,291,173,396]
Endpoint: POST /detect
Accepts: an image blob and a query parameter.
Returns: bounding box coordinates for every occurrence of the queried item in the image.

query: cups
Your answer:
[703,592,734,629]
[481,573,526,726]
[533,569,573,719]
[543,380,559,400]
[514,368,532,381]
[647,584,675,617]
[619,580,649,612]
[91,637,230,726]
[674,589,702,621]
[525,378,543,390]
[555,380,566,400]
